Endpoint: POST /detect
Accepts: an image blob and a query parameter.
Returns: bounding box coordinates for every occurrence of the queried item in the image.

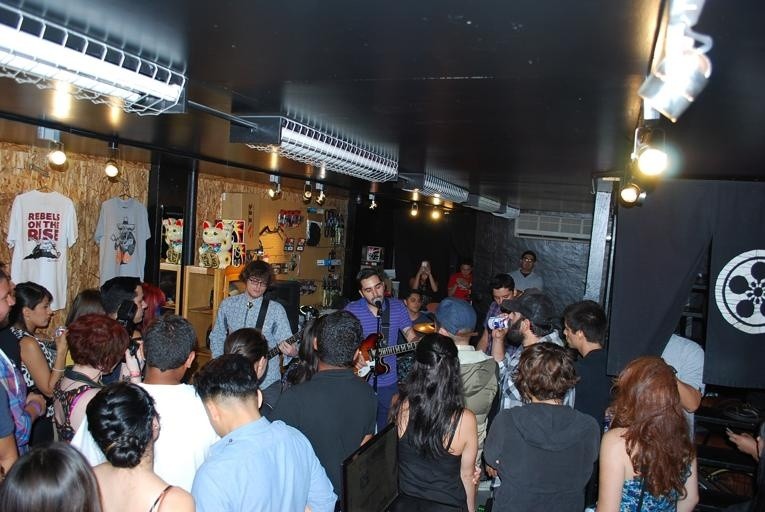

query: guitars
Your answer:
[353,332,419,382]
[267,328,305,362]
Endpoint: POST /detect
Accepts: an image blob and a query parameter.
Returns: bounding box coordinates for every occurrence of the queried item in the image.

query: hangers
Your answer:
[35,173,55,192]
[118,182,133,200]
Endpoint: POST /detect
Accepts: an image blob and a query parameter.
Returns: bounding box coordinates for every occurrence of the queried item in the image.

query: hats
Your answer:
[501,288,555,326]
[426,296,479,337]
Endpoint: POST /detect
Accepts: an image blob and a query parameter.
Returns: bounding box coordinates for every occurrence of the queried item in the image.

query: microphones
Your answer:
[372,298,383,313]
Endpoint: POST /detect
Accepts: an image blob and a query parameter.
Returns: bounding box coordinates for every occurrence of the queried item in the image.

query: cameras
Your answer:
[114,299,140,356]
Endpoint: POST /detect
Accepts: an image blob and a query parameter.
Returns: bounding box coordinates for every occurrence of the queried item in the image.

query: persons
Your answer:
[0,443,104,512]
[0,383,19,475]
[476,273,523,357]
[596,356,699,512]
[402,289,431,324]
[191,354,337,511]
[481,341,600,512]
[10,281,70,450]
[447,260,473,306]
[65,289,106,367]
[661,333,706,444]
[342,268,425,446]
[224,328,268,381]
[426,296,498,490]
[209,260,298,419]
[85,381,196,512]
[409,258,439,305]
[99,277,148,338]
[491,288,576,489]
[0,270,47,457]
[398,332,477,512]
[506,250,544,292]
[726,423,765,469]
[69,316,223,493]
[53,313,145,445]
[269,310,377,512]
[563,300,610,429]
[139,283,165,337]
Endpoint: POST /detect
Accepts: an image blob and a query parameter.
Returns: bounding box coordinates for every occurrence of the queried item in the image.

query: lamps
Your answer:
[636,25,709,125]
[267,172,282,200]
[102,136,122,179]
[409,190,422,218]
[316,182,328,206]
[427,195,441,221]
[618,177,643,206]
[48,138,69,169]
[631,125,670,179]
[302,177,313,203]
[367,192,379,212]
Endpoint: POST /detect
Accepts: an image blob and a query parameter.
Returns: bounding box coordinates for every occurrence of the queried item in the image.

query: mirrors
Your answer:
[150,149,197,317]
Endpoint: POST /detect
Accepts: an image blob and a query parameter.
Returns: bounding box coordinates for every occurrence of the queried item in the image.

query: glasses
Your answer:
[522,256,534,262]
[248,277,267,286]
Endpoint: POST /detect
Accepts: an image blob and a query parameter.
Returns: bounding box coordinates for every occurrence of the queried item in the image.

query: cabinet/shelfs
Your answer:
[183,259,252,357]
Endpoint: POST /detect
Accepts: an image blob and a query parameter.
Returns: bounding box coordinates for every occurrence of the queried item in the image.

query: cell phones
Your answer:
[420,261,427,267]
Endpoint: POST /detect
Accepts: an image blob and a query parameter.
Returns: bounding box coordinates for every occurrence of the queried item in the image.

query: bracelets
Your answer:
[128,372,142,383]
[52,369,66,373]
[29,399,43,416]
